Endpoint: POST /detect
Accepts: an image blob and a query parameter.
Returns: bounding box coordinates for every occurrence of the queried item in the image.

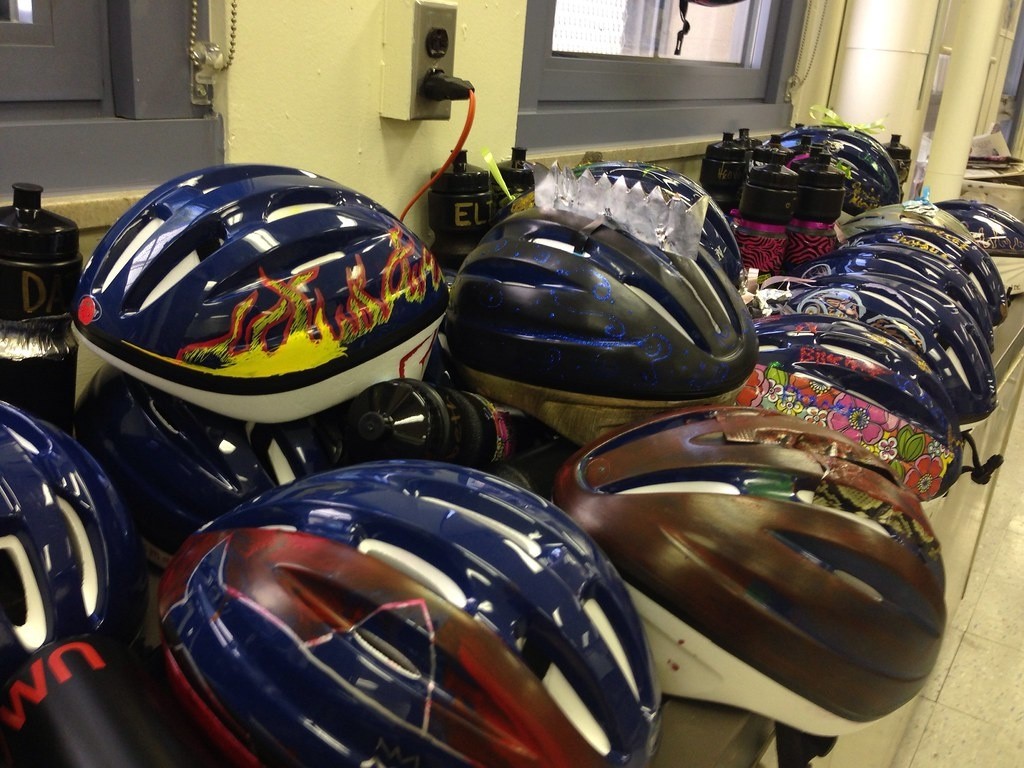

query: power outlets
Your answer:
[410,0,458,121]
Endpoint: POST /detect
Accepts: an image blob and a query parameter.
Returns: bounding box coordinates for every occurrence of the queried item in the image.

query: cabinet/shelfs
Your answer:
[752,345,1023,767]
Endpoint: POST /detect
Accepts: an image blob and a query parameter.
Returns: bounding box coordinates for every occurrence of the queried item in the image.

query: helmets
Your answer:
[72,164,456,436]
[763,123,904,217]
[444,211,762,452]
[71,351,432,571]
[0,401,151,752]
[134,458,659,768]
[554,406,944,732]
[737,197,1024,501]
